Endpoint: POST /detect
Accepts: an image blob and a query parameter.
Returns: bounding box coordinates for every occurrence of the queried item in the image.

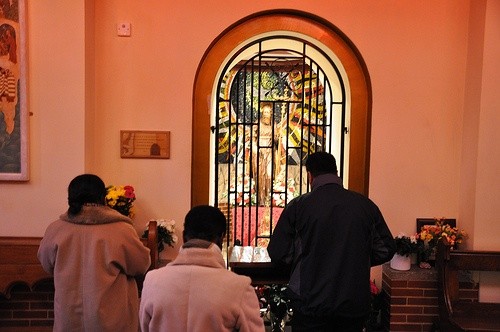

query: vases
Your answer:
[417,249,431,271]
[390,253,411,270]
[260,308,273,332]
[435,246,453,270]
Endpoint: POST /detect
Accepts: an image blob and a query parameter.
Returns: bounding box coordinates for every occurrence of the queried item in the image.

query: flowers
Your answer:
[254,282,293,332]
[394,233,417,257]
[142,218,178,251]
[415,217,464,250]
[104,186,136,222]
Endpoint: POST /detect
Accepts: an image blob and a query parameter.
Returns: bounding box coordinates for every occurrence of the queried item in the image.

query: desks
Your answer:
[452,303,500,332]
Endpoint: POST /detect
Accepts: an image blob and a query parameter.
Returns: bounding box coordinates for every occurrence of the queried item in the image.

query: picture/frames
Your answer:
[0,0,31,183]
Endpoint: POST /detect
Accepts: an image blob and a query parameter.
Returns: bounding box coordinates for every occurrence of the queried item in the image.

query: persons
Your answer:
[37,173,152,332]
[139,205,266,332]
[267,152,395,332]
